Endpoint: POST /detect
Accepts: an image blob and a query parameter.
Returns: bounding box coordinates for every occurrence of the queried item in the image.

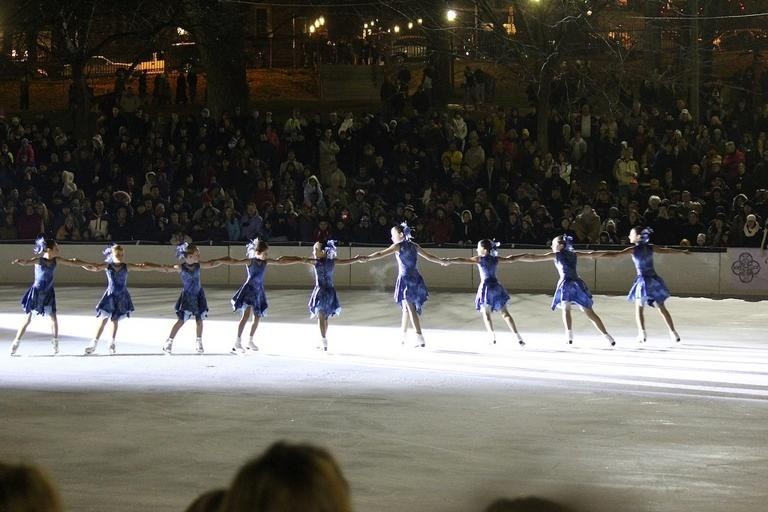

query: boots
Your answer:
[490,333,497,344]
[235,336,258,353]
[9,339,20,352]
[163,337,172,354]
[605,332,615,345]
[640,329,647,342]
[566,329,573,344]
[317,338,328,351]
[196,337,204,353]
[515,332,523,344]
[52,339,60,349]
[86,340,96,354]
[400,331,425,347]
[109,340,116,353]
[672,329,680,342]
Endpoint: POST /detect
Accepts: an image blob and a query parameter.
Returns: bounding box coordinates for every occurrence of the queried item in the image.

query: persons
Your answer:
[137,243,232,354]
[222,440,354,512]
[359,223,451,347]
[10,233,95,355]
[594,226,690,342]
[67,240,167,355]
[0,460,66,511]
[210,237,304,351]
[1,1,767,250]
[442,238,535,345]
[508,234,619,345]
[277,239,368,349]
